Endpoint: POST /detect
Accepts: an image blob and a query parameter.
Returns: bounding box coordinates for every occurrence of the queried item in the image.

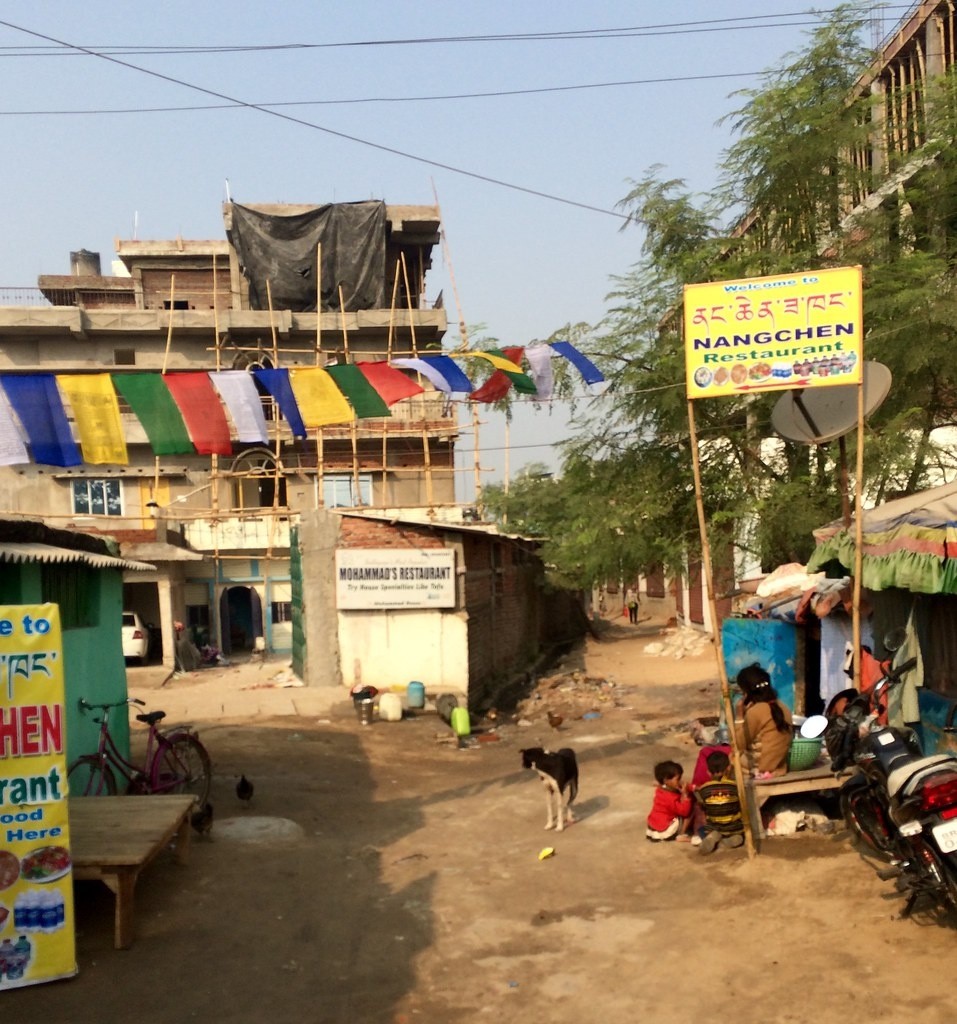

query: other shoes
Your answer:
[677,834,691,842]
[691,834,702,846]
[699,830,722,854]
[717,834,743,849]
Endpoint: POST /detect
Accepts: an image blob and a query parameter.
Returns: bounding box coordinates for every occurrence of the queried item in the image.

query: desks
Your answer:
[71,792,201,948]
[746,761,855,839]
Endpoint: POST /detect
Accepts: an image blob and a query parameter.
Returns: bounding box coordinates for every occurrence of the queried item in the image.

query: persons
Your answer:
[734,664,792,771]
[625,584,640,624]
[696,752,743,854]
[646,761,693,841]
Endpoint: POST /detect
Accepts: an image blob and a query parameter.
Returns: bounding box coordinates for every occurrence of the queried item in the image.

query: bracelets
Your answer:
[735,720,744,723]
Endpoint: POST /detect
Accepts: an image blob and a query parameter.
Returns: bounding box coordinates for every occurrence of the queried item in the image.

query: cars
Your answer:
[121,611,156,665]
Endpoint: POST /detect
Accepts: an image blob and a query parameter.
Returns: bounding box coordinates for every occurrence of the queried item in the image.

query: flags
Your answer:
[551,341,604,384]
[57,372,128,464]
[254,355,472,437]
[165,372,232,456]
[113,372,195,455]
[209,370,269,444]
[452,347,538,402]
[0,375,82,467]
[525,345,552,399]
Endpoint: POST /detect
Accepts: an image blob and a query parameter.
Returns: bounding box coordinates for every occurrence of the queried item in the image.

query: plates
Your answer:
[19,846,71,884]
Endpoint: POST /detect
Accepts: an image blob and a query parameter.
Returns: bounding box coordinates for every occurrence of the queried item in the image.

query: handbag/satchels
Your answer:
[624,607,628,617]
[629,601,635,608]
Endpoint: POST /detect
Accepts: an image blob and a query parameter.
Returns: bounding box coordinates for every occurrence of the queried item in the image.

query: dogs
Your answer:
[517,743,579,832]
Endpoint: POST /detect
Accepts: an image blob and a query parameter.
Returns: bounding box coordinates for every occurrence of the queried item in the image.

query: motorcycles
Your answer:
[800,626,957,917]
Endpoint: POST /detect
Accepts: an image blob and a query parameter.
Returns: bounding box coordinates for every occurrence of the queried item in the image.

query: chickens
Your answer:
[235,773,255,807]
[190,801,214,839]
[547,712,564,729]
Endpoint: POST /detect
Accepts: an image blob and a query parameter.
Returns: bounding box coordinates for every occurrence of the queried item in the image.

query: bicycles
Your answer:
[67,695,214,810]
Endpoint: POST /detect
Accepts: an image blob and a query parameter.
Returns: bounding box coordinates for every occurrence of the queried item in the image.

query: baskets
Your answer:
[786,736,825,772]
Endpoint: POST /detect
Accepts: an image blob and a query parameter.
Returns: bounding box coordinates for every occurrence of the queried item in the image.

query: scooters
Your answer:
[187,626,220,666]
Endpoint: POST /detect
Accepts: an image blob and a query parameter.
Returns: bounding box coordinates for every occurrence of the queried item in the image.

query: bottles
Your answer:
[13,887,65,934]
[0,936,30,983]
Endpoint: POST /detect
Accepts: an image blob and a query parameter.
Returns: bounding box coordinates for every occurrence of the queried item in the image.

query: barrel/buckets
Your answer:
[407,681,425,710]
[354,699,374,726]
[378,693,402,721]
[450,706,471,736]
